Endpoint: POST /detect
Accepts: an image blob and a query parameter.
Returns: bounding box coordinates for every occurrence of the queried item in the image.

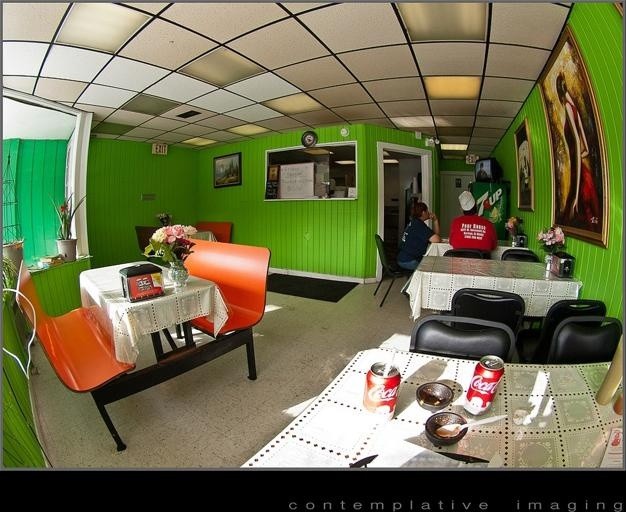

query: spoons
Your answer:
[435,414,510,440]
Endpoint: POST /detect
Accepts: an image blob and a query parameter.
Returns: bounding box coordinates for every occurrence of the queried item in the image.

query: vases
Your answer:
[56,239,78,264]
[511,234,516,247]
[164,257,190,288]
[2,240,24,269]
[544,252,552,271]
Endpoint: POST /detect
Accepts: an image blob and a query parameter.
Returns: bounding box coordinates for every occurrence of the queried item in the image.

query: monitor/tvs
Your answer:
[474,157,503,182]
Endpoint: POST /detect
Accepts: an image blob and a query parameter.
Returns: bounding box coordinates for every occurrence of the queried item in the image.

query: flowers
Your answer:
[534,224,568,254]
[504,215,523,236]
[46,187,90,240]
[140,222,199,263]
[153,210,174,225]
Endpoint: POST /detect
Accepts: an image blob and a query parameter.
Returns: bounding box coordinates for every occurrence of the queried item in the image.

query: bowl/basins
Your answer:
[425,411,469,448]
[416,382,453,413]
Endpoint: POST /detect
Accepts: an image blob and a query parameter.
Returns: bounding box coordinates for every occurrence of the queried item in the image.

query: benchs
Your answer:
[134,226,165,251]
[11,257,135,455]
[194,222,234,242]
[174,235,274,385]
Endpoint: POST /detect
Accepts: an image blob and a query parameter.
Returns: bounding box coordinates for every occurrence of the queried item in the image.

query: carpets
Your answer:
[264,270,362,306]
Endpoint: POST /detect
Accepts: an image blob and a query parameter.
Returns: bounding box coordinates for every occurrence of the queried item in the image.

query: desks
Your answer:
[402,254,590,328]
[76,256,231,369]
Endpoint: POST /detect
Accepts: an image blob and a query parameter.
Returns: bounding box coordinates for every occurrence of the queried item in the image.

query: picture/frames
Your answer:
[266,164,280,182]
[535,22,611,250]
[212,150,245,189]
[612,1,624,23]
[512,114,536,213]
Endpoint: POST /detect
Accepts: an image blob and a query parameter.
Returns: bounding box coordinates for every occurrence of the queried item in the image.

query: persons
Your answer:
[521,156,530,204]
[556,71,600,230]
[449,190,497,259]
[477,163,488,179]
[397,202,441,301]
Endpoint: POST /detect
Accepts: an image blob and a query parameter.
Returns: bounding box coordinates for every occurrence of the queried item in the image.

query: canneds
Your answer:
[462,354,504,415]
[364,362,400,424]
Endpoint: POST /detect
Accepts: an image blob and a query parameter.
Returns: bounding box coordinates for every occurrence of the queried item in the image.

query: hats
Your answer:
[458,190,476,211]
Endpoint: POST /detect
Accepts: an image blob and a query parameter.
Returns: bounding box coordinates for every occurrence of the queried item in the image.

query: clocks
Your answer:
[301,131,318,149]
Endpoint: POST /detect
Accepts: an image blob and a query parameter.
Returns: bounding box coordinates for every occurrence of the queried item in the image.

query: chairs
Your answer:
[544,315,622,364]
[373,232,414,308]
[448,282,525,345]
[442,248,485,260]
[500,248,541,263]
[514,296,607,363]
[408,312,518,364]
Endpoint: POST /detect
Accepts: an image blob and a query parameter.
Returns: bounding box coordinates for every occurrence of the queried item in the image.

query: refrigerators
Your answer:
[467,182,509,240]
